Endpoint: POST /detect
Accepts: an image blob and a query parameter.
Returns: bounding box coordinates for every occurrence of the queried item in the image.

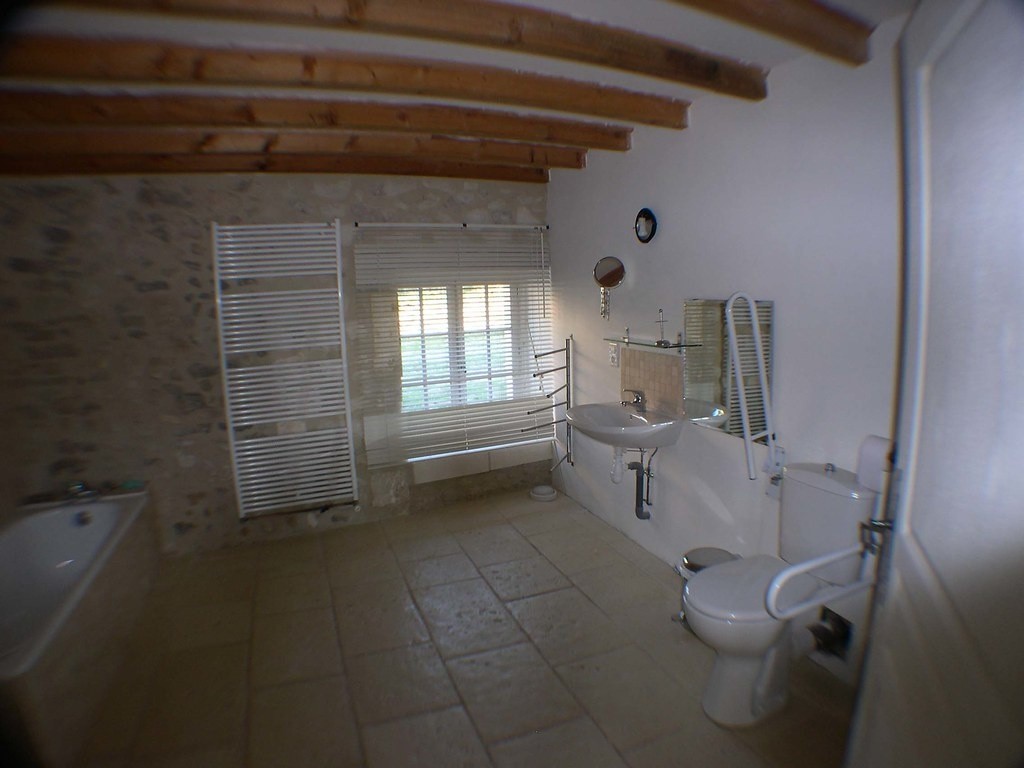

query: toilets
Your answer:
[681,462,877,729]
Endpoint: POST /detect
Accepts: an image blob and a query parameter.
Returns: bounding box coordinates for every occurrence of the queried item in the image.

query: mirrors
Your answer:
[593,256,626,289]
[635,207,657,243]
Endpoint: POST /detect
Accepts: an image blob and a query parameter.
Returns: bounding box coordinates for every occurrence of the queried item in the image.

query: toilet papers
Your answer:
[857,435,894,491]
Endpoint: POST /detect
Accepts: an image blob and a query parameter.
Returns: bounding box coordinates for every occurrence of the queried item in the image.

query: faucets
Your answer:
[619,388,646,412]
[67,481,84,496]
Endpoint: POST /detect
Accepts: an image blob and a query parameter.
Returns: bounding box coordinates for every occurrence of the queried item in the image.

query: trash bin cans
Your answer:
[672,547,744,633]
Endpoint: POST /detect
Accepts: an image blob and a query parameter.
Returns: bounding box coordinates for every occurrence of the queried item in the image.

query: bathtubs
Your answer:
[0,492,156,768]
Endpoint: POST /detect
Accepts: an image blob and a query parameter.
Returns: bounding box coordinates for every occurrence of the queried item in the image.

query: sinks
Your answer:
[563,401,682,448]
[671,399,731,429]
